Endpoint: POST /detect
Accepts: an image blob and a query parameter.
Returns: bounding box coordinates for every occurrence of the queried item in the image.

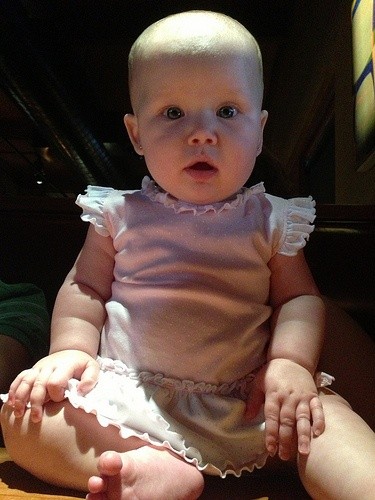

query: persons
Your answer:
[1,10,375,500]
